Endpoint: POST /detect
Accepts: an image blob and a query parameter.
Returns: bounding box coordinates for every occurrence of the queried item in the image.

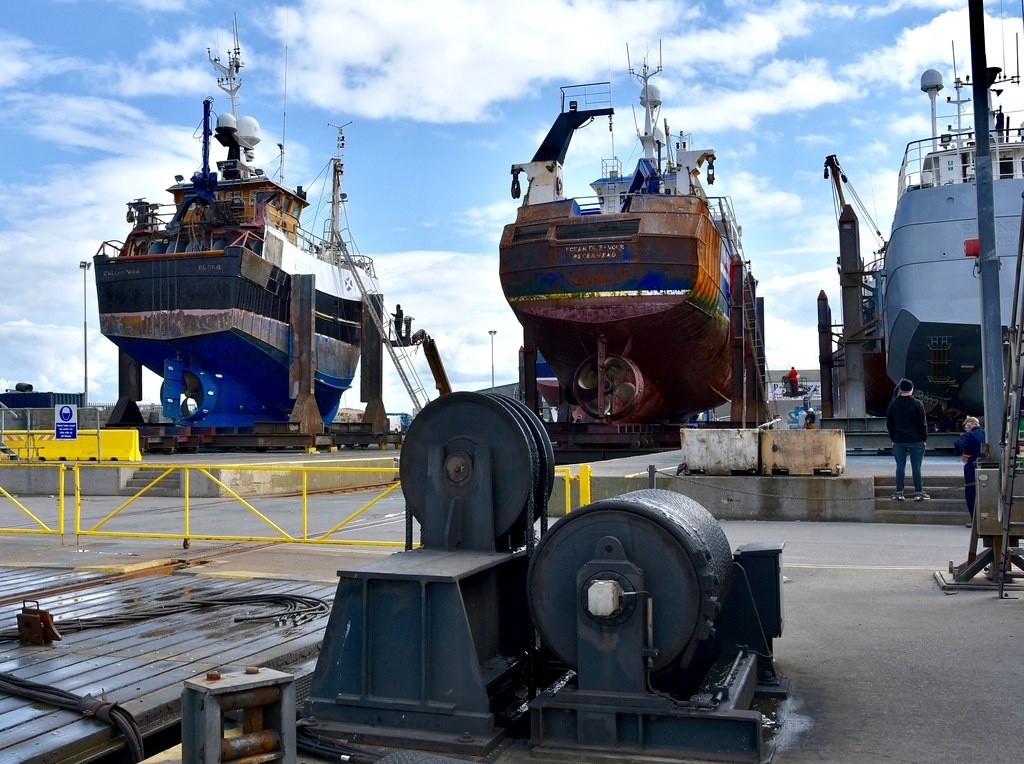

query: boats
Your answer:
[876,2,1024,417]
[93,19,381,428]
[500,38,764,424]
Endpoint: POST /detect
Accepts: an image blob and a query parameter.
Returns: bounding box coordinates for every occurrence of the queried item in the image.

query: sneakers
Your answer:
[892,490,905,500]
[914,491,930,500]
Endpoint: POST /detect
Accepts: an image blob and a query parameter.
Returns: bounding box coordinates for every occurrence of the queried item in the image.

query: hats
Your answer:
[808,408,813,411]
[899,379,913,392]
[962,417,979,425]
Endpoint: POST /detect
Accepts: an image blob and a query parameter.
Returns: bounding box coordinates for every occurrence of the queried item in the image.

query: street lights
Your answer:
[79,260,91,406]
[487,331,498,395]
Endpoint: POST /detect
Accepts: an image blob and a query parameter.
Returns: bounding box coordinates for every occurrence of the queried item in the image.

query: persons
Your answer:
[805,408,816,429]
[787,367,798,391]
[886,379,930,501]
[390,304,403,339]
[954,417,986,528]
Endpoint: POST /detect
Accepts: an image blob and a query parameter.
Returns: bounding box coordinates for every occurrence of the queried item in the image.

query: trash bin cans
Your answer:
[781,376,794,397]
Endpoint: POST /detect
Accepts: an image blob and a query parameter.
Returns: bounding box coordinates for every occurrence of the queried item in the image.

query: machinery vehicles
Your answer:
[382,328,453,395]
[824,153,888,255]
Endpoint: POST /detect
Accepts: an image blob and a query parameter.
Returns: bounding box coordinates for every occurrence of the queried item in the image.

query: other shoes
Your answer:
[966,523,972,528]
